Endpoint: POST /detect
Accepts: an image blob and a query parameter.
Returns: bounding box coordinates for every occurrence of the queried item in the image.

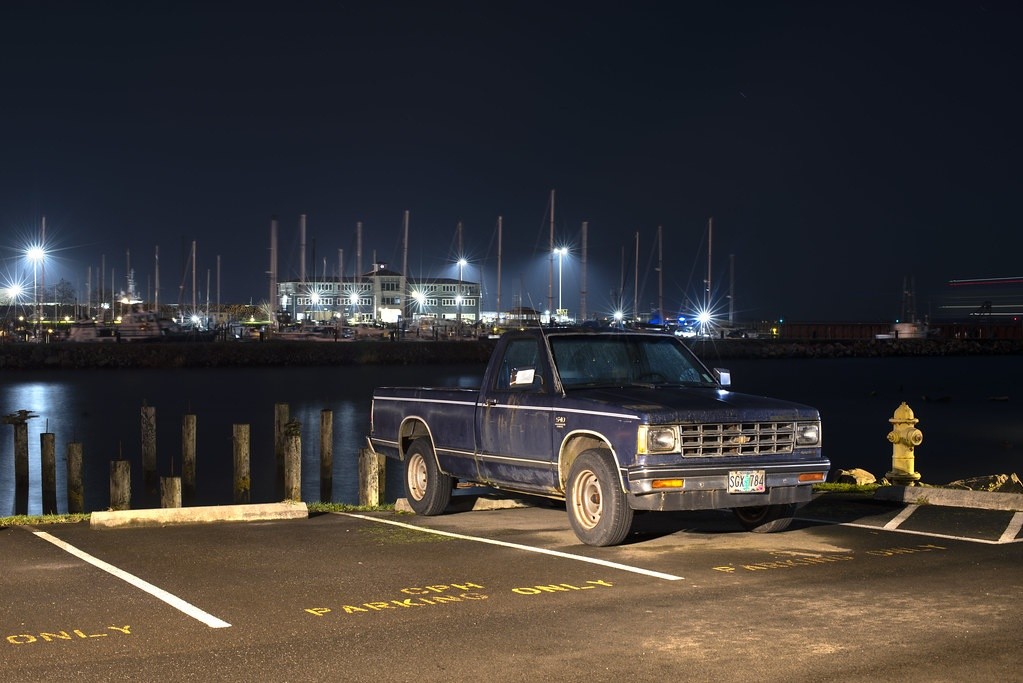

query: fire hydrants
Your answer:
[885,401,923,487]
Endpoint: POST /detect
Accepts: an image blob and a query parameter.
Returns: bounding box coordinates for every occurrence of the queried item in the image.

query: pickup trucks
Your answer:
[368,326,832,549]
